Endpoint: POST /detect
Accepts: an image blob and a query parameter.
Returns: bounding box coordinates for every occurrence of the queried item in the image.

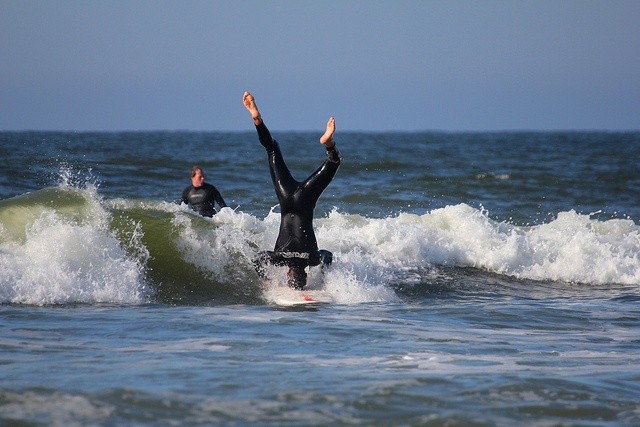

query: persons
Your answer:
[178,166,227,218]
[241,90,343,291]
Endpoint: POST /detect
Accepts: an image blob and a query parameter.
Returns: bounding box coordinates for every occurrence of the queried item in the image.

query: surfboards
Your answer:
[271,289,332,305]
